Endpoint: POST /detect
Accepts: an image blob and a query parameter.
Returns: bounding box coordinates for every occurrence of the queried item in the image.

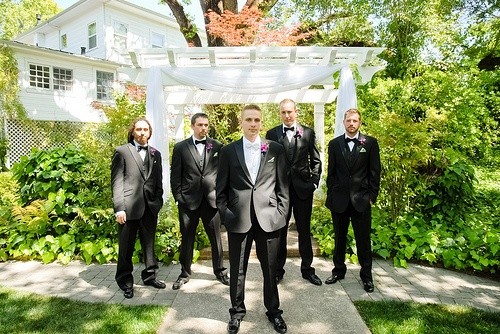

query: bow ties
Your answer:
[195,140,207,145]
[346,137,356,143]
[246,142,259,151]
[284,127,294,131]
[137,146,148,152]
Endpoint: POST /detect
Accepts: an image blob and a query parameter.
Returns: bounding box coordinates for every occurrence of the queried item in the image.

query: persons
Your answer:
[265,98,323,286]
[325,109,381,293]
[170,113,230,290]
[110,119,166,298]
[216,104,291,334]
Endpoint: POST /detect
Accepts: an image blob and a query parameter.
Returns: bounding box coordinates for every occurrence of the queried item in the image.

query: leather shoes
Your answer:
[124,288,133,298]
[363,281,374,293]
[325,274,344,284]
[228,319,240,333]
[144,278,166,288]
[302,273,322,284]
[276,275,283,284]
[268,315,287,333]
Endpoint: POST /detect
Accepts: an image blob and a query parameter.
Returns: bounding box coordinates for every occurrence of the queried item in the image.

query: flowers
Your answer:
[260,144,269,153]
[359,136,366,146]
[150,149,156,156]
[296,127,303,138]
[207,143,212,149]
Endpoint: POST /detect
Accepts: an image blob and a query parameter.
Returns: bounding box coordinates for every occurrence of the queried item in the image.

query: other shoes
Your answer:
[173,277,189,290]
[216,275,230,285]
